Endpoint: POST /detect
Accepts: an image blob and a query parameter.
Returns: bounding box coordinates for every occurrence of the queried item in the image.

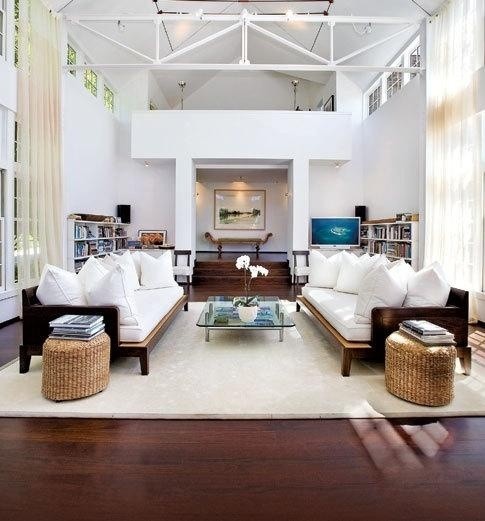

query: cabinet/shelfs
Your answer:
[68,218,129,274]
[360,221,419,271]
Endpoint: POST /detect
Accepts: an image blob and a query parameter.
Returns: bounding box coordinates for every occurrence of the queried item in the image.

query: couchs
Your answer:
[19,249,188,375]
[296,251,472,376]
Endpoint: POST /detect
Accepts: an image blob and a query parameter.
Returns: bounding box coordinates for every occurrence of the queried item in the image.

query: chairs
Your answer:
[172,250,191,292]
[293,251,310,293]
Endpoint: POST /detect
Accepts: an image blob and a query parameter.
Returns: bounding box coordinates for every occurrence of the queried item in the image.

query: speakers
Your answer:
[354,206,365,224]
[117,205,131,224]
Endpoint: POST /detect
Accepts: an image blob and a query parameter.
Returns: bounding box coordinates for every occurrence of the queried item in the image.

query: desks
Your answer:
[204,232,273,259]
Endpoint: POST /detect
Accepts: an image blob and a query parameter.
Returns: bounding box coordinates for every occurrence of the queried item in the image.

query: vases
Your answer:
[238,304,258,323]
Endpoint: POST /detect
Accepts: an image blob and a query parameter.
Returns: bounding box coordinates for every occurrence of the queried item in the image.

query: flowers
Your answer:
[234,254,268,303]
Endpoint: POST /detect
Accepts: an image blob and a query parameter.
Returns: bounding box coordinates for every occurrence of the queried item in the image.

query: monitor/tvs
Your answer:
[310,217,360,250]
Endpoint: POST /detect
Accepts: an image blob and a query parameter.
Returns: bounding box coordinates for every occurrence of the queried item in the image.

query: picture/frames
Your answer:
[214,189,266,230]
[138,229,167,245]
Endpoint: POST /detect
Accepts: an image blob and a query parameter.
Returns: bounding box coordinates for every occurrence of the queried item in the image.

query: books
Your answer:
[373,226,386,238]
[74,225,93,239]
[388,241,411,258]
[389,225,410,239]
[401,320,455,346]
[116,238,128,249]
[75,241,97,257]
[373,241,388,253]
[97,226,113,238]
[98,240,113,253]
[50,315,105,342]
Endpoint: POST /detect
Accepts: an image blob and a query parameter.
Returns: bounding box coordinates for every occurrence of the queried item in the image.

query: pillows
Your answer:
[139,251,178,288]
[36,263,87,306]
[404,261,452,307]
[333,251,367,294]
[389,258,414,285]
[340,251,403,263]
[80,255,137,325]
[115,250,140,291]
[310,250,338,288]
[355,264,407,324]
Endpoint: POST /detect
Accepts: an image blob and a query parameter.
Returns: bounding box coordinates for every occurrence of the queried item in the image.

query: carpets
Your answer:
[0,301,484,418]
